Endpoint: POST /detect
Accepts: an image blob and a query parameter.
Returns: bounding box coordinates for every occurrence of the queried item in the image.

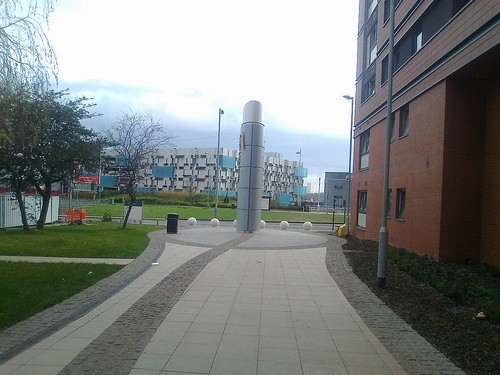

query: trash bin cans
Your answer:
[166,212,180,235]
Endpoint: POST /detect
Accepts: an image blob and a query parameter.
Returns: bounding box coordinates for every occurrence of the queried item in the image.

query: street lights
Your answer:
[342,95,354,237]
[214,109,226,218]
